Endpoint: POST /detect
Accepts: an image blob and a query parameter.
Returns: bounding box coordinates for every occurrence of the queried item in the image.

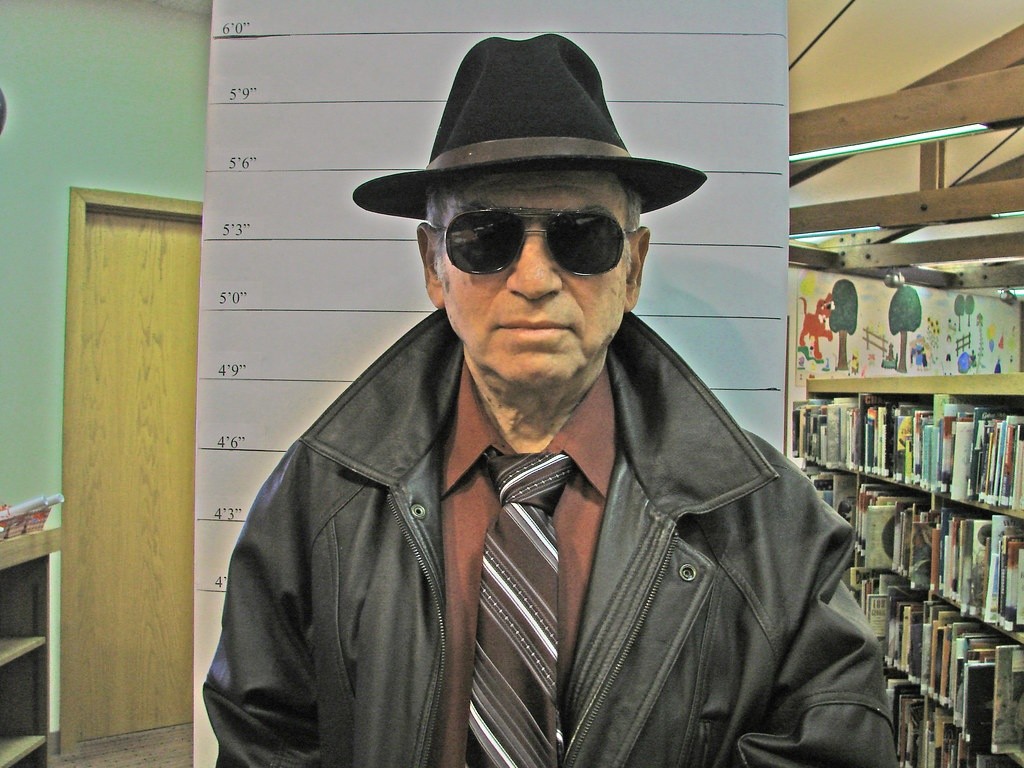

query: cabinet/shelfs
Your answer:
[795,379,1024,768]
[0,525,62,768]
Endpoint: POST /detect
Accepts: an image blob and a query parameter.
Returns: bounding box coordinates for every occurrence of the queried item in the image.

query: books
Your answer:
[770,468,1024,638]
[789,391,1022,511]
[832,568,1024,768]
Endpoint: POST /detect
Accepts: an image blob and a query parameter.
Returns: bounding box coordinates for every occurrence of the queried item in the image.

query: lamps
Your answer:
[884,267,905,288]
[997,286,1018,306]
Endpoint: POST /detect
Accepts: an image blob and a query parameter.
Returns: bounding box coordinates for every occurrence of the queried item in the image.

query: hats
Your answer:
[351,34,709,221]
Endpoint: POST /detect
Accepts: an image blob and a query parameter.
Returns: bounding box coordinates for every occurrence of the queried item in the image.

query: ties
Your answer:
[464,450,578,768]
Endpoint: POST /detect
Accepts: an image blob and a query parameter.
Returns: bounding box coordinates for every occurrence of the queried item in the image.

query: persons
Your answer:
[199,35,902,768]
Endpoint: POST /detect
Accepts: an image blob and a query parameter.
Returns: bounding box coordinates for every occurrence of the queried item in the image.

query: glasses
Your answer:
[432,207,637,276]
